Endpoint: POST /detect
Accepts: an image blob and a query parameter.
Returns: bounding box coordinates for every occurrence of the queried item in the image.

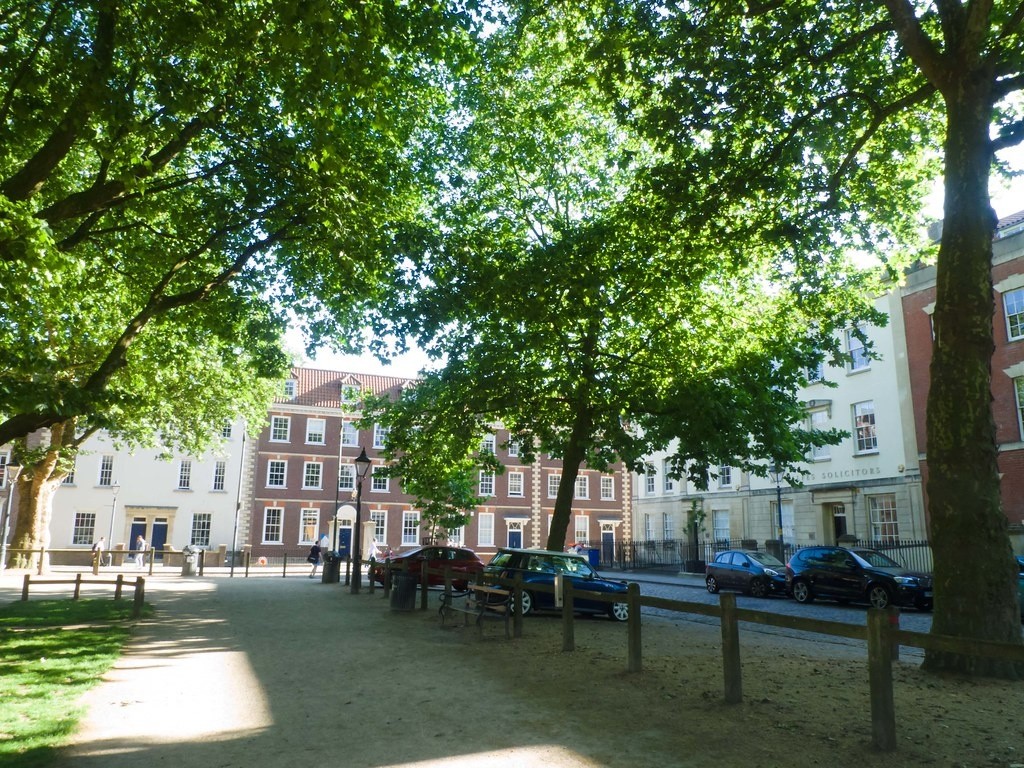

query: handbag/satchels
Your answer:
[92,544,96,550]
[307,555,317,564]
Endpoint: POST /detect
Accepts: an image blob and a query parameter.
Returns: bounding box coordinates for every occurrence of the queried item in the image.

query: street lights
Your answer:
[106,479,121,550]
[768,461,785,561]
[350,446,373,589]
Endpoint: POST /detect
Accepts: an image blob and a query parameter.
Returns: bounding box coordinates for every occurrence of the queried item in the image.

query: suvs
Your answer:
[785,547,933,613]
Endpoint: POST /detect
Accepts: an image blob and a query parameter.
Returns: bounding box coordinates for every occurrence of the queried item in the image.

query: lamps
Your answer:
[897,464,915,482]
[734,484,750,495]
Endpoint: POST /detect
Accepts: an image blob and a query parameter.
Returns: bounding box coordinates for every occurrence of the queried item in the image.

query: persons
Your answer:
[307,541,323,578]
[572,543,587,554]
[89,536,108,567]
[368,537,394,580]
[134,534,146,570]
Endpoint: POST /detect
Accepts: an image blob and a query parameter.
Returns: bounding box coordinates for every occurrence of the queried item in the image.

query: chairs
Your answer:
[531,560,540,569]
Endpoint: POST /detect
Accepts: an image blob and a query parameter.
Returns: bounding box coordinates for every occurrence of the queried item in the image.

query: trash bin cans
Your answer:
[241,544,252,567]
[389,573,418,612]
[322,550,340,582]
[588,548,603,571]
[182,544,203,577]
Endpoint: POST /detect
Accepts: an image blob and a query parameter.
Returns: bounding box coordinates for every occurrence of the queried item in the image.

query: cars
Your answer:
[480,547,630,623]
[1015,555,1024,624]
[705,549,793,599]
[367,544,486,592]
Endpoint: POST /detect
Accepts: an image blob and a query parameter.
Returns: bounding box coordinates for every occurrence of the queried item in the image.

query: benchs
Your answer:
[439,584,512,639]
